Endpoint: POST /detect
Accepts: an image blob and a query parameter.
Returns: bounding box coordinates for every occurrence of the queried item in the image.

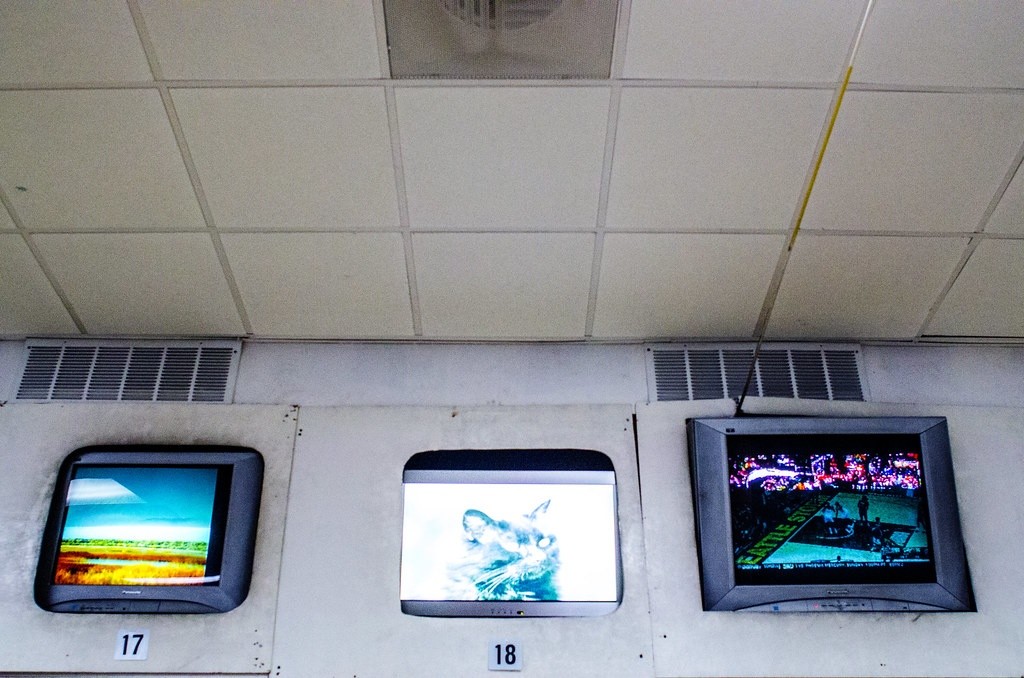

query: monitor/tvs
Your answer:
[687,416,978,612]
[41,451,261,614]
[400,470,622,618]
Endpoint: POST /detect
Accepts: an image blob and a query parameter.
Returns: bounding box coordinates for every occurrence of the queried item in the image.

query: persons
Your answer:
[729,452,924,563]
